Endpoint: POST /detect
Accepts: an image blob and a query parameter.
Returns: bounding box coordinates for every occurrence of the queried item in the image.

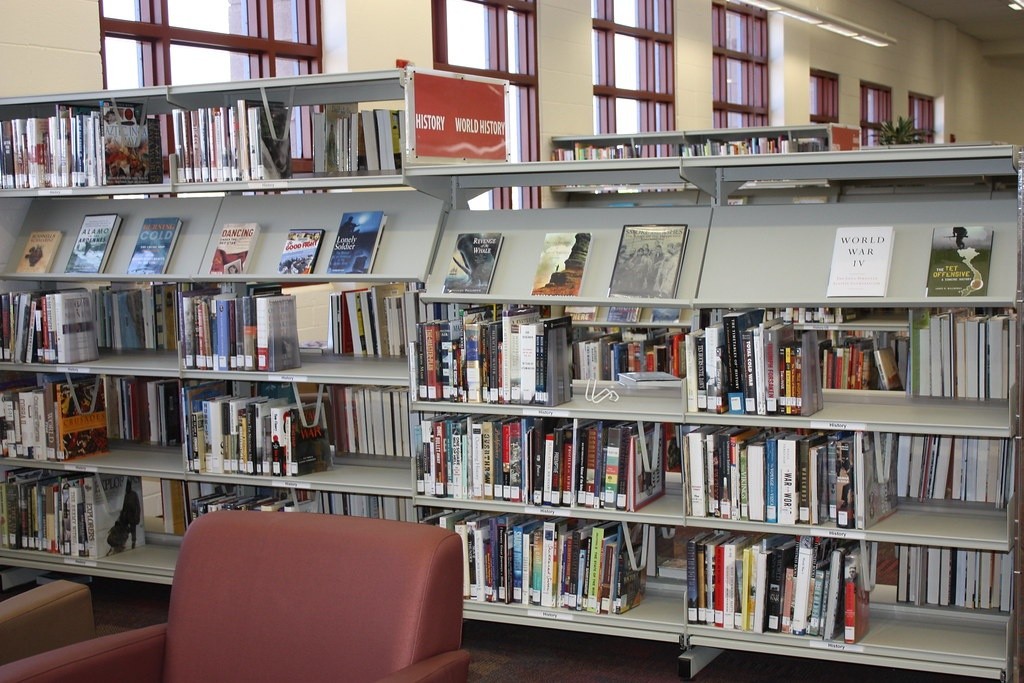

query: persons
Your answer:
[845,566,858,579]
[122,481,141,550]
[107,520,128,555]
[228,265,238,275]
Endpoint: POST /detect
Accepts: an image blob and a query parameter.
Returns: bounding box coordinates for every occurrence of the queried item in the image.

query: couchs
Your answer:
[0,580,95,666]
[0,511,469,683]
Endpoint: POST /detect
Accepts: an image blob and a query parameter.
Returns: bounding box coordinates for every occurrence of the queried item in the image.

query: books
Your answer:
[0,99,1014,645]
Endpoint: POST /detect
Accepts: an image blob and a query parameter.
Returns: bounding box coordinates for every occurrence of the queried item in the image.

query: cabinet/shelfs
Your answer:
[551,122,862,160]
[404,145,1024,683]
[0,64,510,585]
